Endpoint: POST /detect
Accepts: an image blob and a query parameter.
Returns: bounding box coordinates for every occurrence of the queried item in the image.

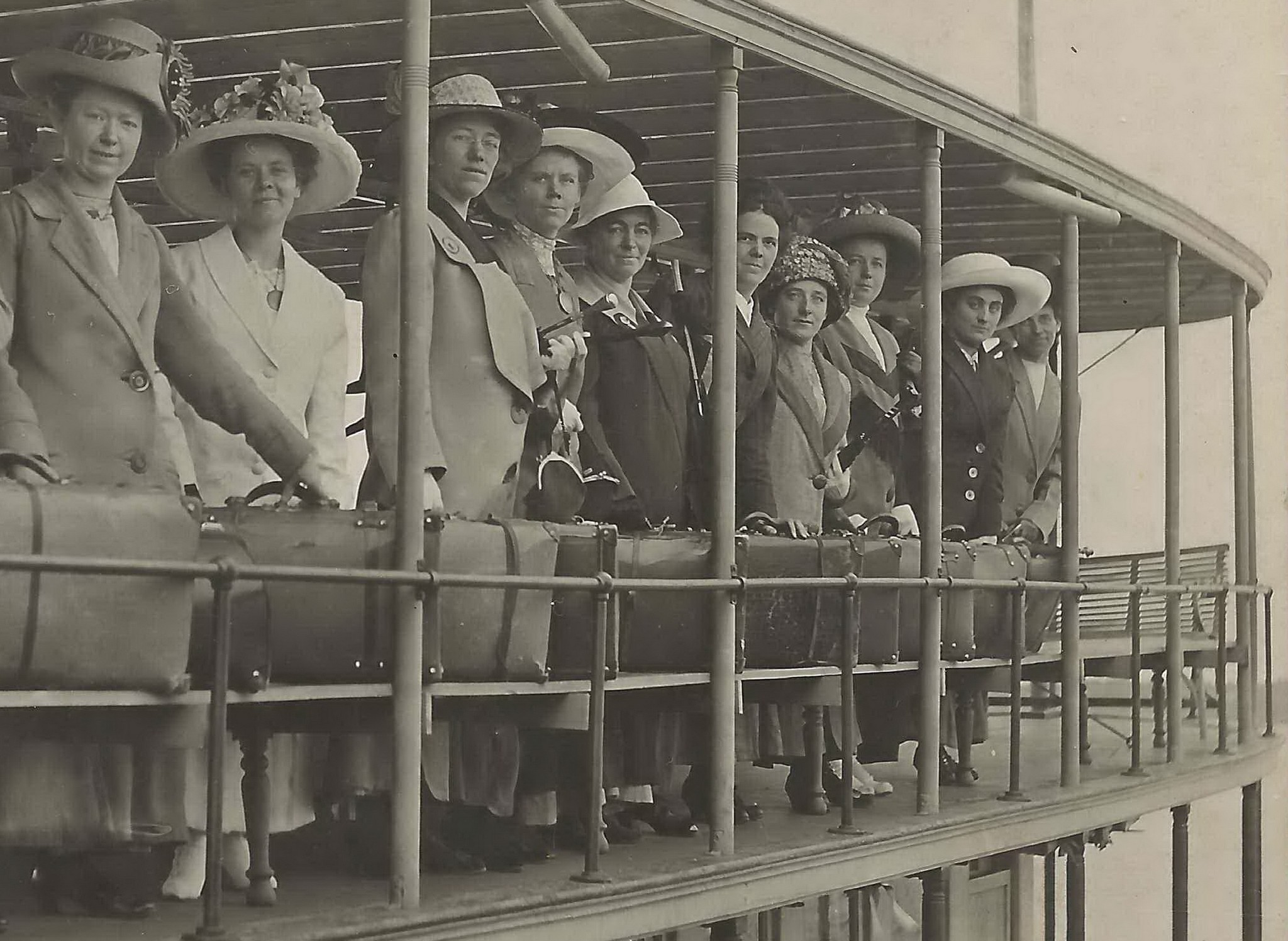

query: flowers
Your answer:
[189,77,259,132]
[260,65,334,128]
[157,38,197,140]
[502,92,539,118]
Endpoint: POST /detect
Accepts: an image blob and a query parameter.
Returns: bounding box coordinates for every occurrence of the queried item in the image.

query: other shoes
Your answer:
[1,728,982,921]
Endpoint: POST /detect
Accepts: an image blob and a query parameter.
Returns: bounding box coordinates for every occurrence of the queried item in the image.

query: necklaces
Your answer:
[234,233,283,311]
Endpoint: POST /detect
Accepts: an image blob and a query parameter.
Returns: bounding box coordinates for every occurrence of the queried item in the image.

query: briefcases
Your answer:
[963,535,1031,660]
[205,481,445,685]
[437,512,562,688]
[180,485,274,695]
[614,519,747,674]
[546,516,620,681]
[897,524,978,663]
[851,514,902,665]
[732,512,864,667]
[1024,541,1062,657]
[1,453,202,695]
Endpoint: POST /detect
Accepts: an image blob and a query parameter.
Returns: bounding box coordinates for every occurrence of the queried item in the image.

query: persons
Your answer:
[336,61,1066,875]
[0,16,331,916]
[142,58,363,899]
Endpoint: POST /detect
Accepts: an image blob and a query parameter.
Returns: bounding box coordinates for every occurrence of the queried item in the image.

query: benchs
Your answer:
[0,635,1235,941]
[1026,542,1233,742]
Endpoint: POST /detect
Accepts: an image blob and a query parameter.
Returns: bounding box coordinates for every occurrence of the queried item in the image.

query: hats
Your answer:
[812,189,922,301]
[480,103,650,229]
[905,252,1052,331]
[9,17,191,164]
[569,171,683,245]
[376,64,544,185]
[153,59,363,222]
[756,234,852,329]
